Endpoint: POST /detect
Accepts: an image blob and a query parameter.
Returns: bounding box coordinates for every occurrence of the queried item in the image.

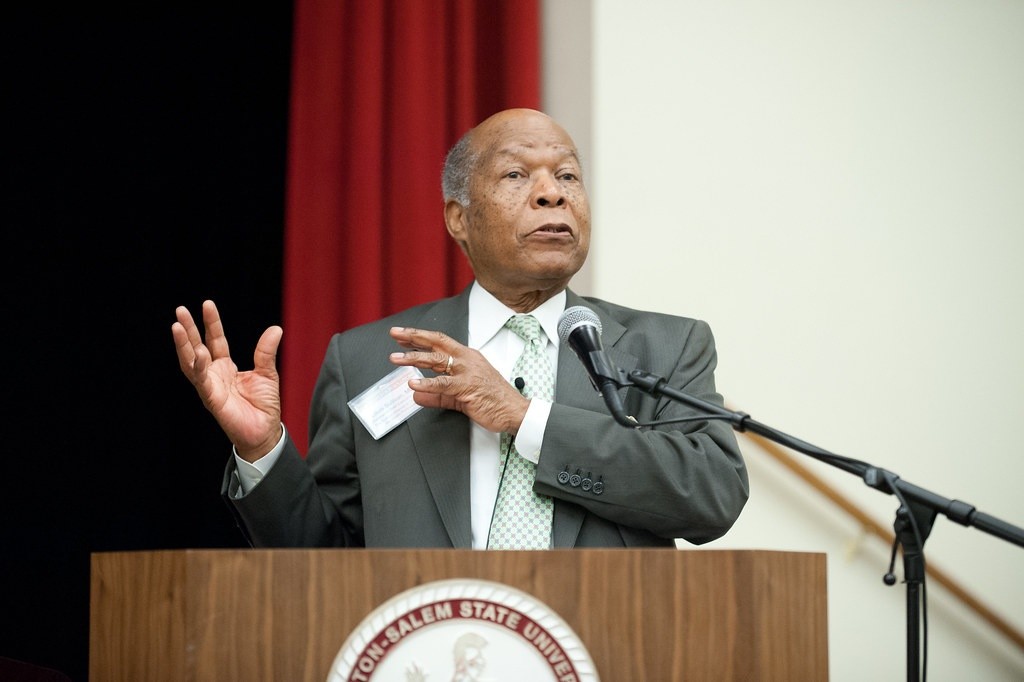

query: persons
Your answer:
[170,107,753,551]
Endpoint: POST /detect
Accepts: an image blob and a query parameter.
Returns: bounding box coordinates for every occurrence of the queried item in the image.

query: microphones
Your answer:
[515,376,526,391]
[558,308,639,427]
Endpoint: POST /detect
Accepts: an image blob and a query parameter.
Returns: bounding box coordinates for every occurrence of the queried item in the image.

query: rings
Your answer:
[446,355,454,374]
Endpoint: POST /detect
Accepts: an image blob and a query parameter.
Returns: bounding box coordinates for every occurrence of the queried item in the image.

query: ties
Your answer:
[487,315,556,549]
